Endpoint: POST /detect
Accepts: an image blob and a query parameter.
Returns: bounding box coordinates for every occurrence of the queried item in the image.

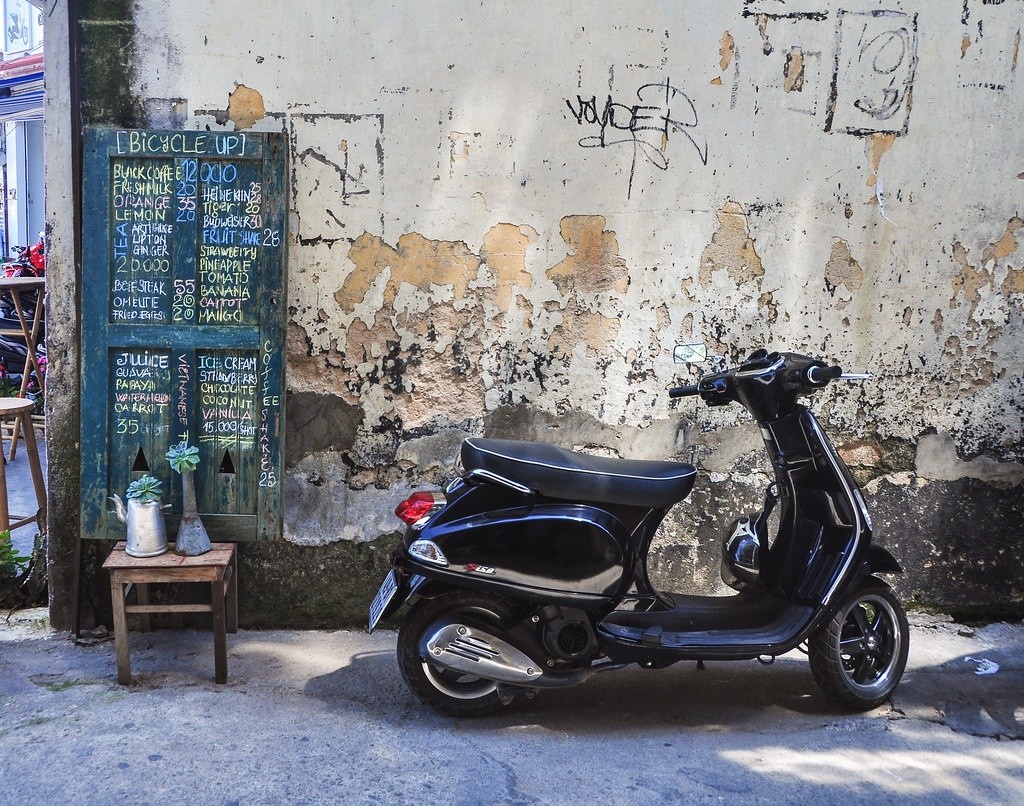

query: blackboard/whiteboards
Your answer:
[76,124,291,542]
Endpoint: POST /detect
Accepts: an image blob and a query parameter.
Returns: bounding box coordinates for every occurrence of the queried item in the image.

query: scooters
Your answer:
[368,347,913,716]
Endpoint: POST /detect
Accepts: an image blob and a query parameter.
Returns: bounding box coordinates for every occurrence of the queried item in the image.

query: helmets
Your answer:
[720,512,770,597]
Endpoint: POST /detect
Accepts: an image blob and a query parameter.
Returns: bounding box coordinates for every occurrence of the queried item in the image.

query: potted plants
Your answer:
[109,473,172,558]
[164,440,212,557]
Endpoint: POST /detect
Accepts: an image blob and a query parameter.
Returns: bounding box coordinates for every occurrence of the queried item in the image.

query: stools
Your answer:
[104,540,239,685]
[0,397,48,541]
